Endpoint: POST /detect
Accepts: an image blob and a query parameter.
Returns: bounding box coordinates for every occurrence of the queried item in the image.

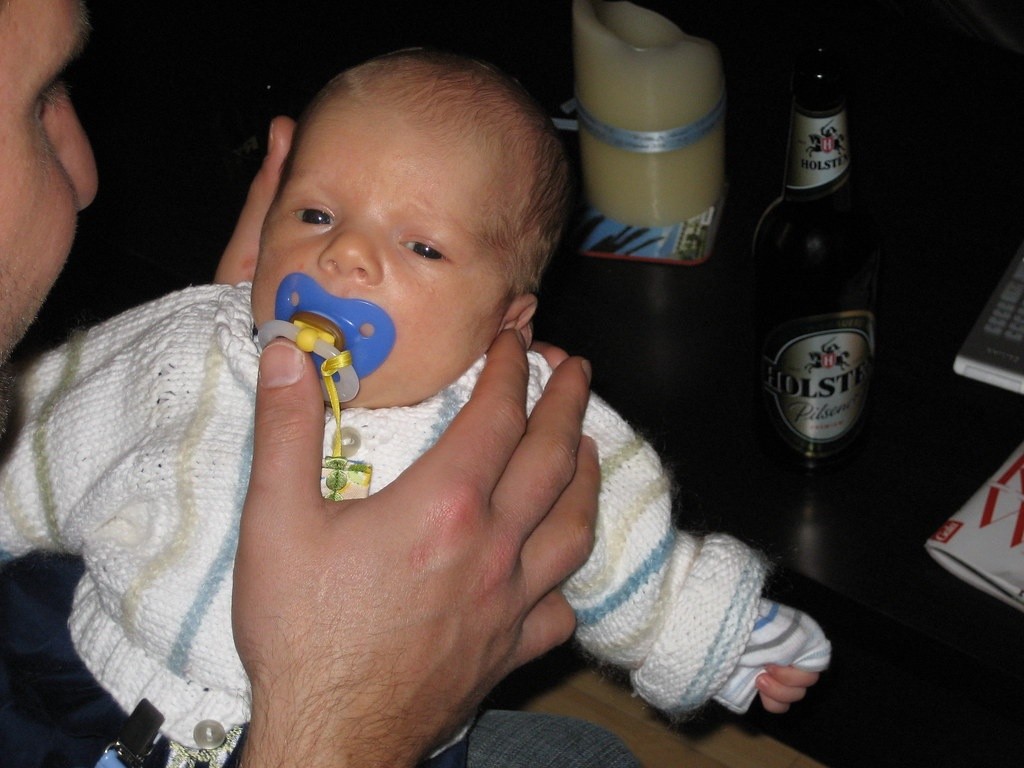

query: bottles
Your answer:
[753,39,880,476]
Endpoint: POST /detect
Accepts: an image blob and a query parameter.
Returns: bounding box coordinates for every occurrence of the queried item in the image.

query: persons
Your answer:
[0,0,641,768]
[0,43,831,768]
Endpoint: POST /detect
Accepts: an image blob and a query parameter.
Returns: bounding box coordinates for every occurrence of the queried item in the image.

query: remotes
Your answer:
[953,242,1024,396]
